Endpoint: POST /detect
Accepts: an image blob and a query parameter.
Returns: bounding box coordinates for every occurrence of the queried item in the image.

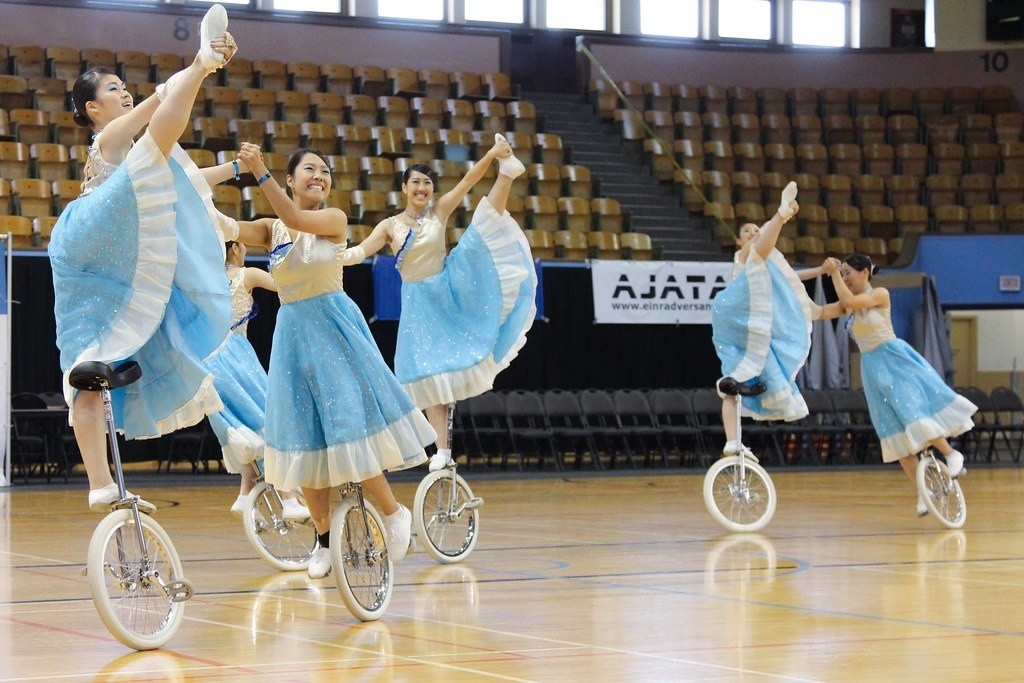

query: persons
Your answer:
[216,141,438,580]
[207,240,311,523]
[813,253,978,514]
[712,181,842,464]
[343,133,538,474]
[49,4,264,516]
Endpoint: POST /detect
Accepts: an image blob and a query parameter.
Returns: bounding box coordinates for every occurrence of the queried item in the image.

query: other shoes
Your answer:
[232,496,267,528]
[945,450,973,481]
[385,505,412,565]
[493,132,528,180]
[426,452,448,474]
[722,443,763,464]
[89,483,158,516]
[307,545,333,580]
[282,499,325,524]
[778,180,802,223]
[198,3,232,73]
[914,489,938,516]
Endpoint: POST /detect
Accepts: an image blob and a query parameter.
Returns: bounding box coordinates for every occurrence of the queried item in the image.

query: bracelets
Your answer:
[258,173,271,185]
[232,160,240,181]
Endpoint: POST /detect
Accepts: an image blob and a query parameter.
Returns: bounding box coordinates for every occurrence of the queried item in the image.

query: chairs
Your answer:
[0,44,1024,269]
[11,387,1024,484]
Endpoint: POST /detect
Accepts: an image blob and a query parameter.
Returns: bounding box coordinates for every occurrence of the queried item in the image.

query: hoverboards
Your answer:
[234,461,324,575]
[412,398,484,563]
[703,376,777,534]
[327,479,395,623]
[914,443,967,530]
[68,360,192,652]
